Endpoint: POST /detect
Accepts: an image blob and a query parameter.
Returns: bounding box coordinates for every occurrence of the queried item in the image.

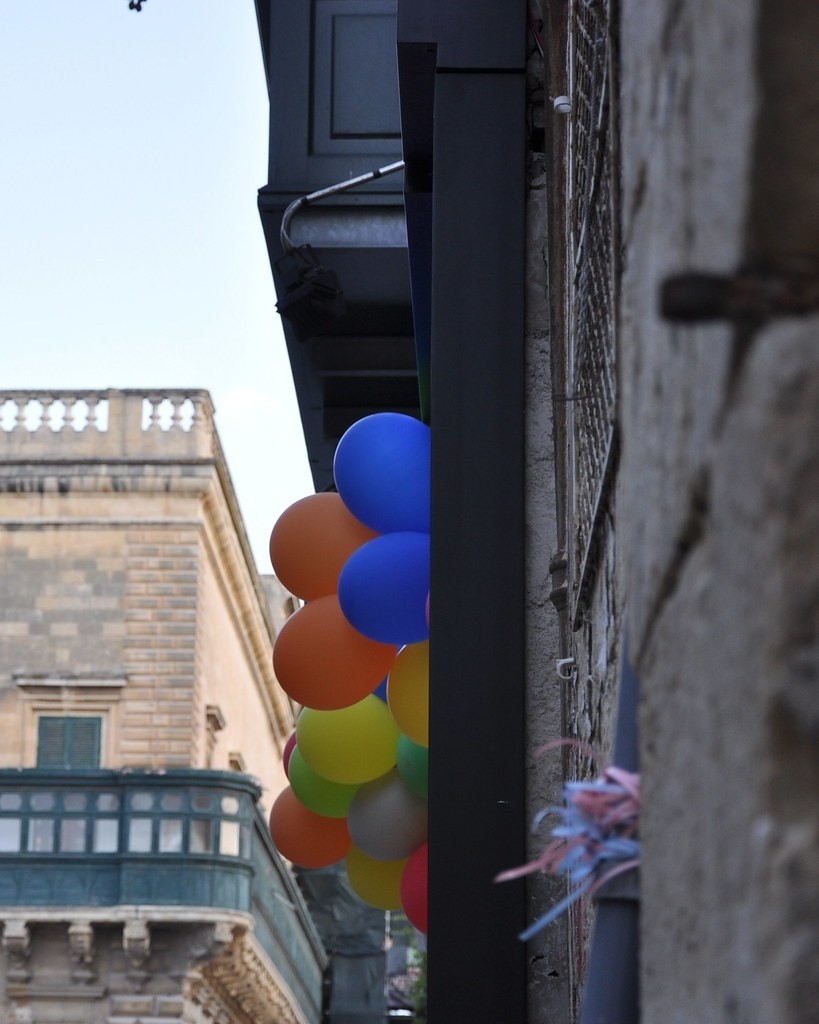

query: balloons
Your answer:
[295,694,399,784]
[337,532,430,645]
[400,843,428,935]
[269,731,428,869]
[346,841,407,911]
[334,412,431,534]
[272,493,427,749]
